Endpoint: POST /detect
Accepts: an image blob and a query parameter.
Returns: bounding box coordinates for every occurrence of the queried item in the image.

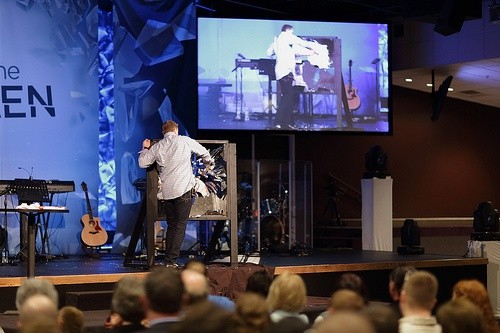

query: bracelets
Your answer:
[144,146,149,150]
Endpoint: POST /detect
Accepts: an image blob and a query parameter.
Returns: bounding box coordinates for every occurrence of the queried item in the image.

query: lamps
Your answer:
[397,219,426,255]
[363,144,389,177]
[469,199,500,241]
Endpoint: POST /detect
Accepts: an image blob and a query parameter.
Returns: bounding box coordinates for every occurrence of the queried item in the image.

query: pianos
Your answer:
[235,58,276,122]
[0,178,76,264]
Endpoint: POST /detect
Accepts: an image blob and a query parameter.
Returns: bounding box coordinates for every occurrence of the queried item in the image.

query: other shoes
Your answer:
[166,263,184,269]
[276,122,298,130]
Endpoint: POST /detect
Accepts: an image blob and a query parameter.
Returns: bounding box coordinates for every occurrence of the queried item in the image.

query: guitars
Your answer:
[341,59,361,112]
[80,181,109,247]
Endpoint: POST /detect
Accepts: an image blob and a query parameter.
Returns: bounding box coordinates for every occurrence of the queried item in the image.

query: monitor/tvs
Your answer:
[194,13,394,137]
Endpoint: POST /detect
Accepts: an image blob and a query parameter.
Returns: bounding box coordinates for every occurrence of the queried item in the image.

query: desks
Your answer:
[0,207,71,281]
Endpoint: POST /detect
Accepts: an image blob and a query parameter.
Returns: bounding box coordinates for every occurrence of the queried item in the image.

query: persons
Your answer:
[268,25,324,129]
[14,263,500,333]
[138,121,213,268]
